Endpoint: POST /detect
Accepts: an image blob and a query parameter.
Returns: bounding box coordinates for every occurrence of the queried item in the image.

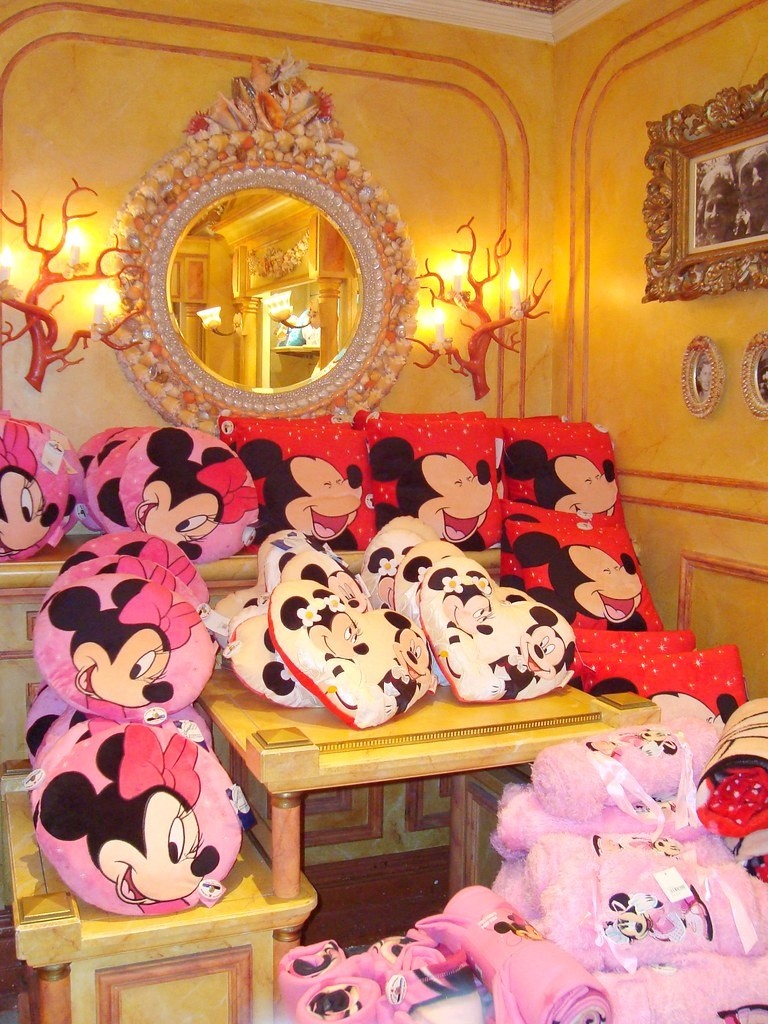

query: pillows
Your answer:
[504,519,666,632]
[500,499,619,595]
[420,557,576,703]
[392,544,538,686]
[571,629,746,742]
[228,538,371,647]
[222,552,373,707]
[211,528,330,650]
[360,530,425,612]
[268,581,438,730]
[504,422,627,531]
[219,415,350,447]
[361,516,441,574]
[233,427,375,551]
[0,410,257,917]
[368,419,504,551]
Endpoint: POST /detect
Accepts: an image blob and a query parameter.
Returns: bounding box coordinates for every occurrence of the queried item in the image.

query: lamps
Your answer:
[196,306,235,336]
[260,290,310,330]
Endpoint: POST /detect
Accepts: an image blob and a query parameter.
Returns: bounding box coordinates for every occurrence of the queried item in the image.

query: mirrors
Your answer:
[102,47,419,438]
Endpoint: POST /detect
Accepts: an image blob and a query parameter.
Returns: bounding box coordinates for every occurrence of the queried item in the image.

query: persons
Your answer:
[696,144,768,244]
[696,355,711,399]
[759,350,768,401]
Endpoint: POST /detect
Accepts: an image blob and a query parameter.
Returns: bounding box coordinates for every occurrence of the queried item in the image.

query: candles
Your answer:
[433,306,446,342]
[91,287,115,325]
[450,254,468,291]
[508,269,521,311]
[0,249,11,282]
[67,226,83,266]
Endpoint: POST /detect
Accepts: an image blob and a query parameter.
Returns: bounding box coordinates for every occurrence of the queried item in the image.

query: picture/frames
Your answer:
[641,72,768,303]
[682,336,728,418]
[742,330,768,421]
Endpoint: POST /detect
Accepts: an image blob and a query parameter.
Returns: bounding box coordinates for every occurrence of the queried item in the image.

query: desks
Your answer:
[194,665,660,900]
[6,790,317,1024]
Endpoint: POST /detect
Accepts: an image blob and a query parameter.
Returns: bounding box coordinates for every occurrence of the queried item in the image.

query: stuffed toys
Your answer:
[274,310,320,346]
